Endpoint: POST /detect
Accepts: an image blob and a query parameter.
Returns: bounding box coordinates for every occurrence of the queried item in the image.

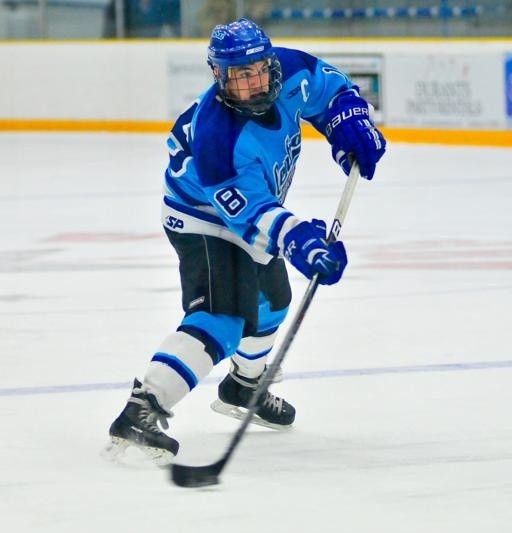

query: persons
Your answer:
[110,16,386,454]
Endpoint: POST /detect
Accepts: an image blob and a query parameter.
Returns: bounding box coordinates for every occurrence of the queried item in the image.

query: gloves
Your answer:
[319,96,387,181]
[279,217,349,287]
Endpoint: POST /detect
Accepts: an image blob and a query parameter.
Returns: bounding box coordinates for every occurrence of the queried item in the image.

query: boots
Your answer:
[217,358,296,427]
[108,376,181,458]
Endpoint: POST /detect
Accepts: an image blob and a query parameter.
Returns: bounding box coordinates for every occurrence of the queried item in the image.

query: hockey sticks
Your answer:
[170,162,359,487]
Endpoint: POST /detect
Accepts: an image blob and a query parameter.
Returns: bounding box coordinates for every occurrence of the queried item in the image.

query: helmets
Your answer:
[205,18,283,118]
